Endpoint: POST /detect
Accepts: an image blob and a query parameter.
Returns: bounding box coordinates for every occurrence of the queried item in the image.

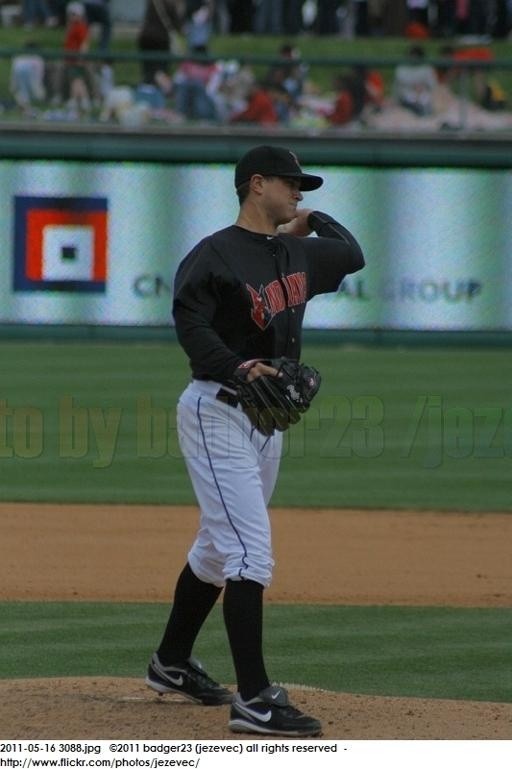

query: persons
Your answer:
[145,146,366,735]
[0,0,512,134]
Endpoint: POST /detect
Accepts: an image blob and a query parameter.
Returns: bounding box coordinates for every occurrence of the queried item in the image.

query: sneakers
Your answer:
[145,652,234,706]
[229,685,322,737]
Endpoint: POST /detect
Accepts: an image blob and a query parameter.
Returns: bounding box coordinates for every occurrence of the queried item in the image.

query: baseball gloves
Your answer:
[231,357,319,435]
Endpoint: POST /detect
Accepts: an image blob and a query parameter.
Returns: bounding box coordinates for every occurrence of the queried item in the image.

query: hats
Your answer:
[235,146,323,191]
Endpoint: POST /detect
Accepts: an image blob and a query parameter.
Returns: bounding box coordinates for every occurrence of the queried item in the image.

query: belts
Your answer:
[216,388,237,407]
